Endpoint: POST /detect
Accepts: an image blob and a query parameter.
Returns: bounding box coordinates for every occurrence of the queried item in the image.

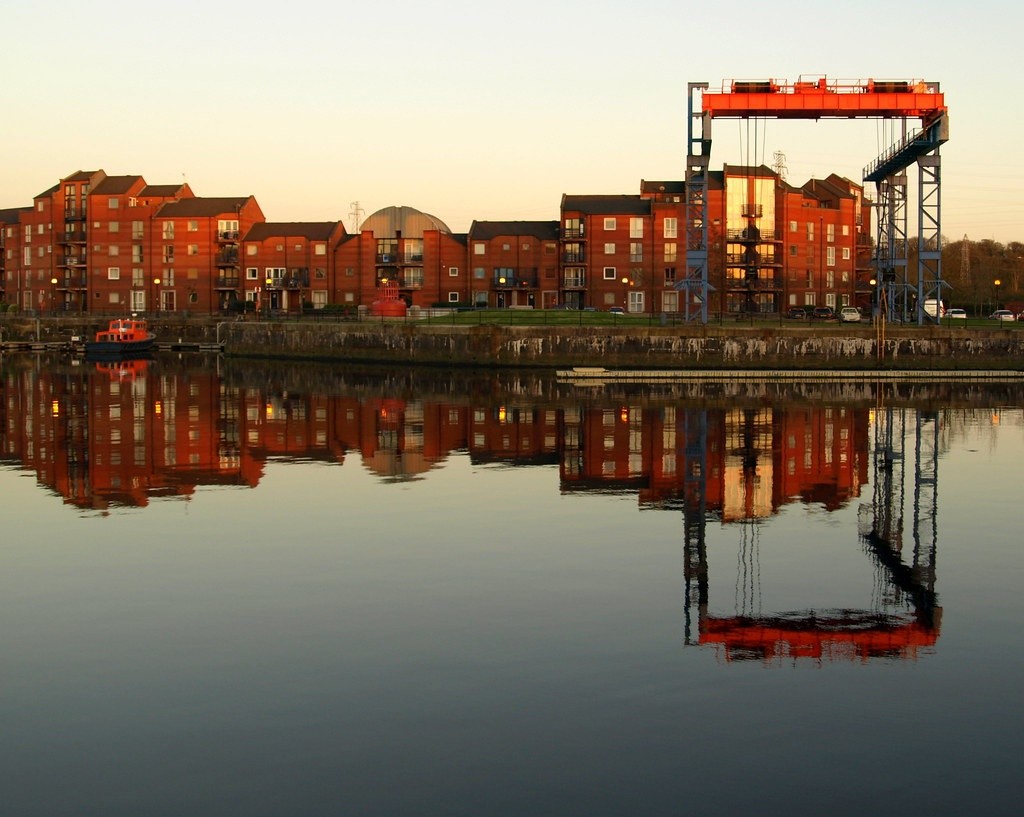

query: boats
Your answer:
[92,354,153,382]
[84,313,157,353]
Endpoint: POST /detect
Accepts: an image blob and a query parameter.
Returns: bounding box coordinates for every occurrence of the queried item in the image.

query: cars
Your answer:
[840,307,863,323]
[813,306,835,319]
[943,307,968,320]
[787,306,806,319]
[584,307,626,314]
[987,309,1024,322]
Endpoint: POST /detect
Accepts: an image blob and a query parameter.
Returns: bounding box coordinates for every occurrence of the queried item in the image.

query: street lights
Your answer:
[154,278,161,312]
[265,278,273,317]
[994,278,1001,322]
[499,276,506,313]
[621,276,629,316]
[51,277,58,313]
[870,279,877,322]
